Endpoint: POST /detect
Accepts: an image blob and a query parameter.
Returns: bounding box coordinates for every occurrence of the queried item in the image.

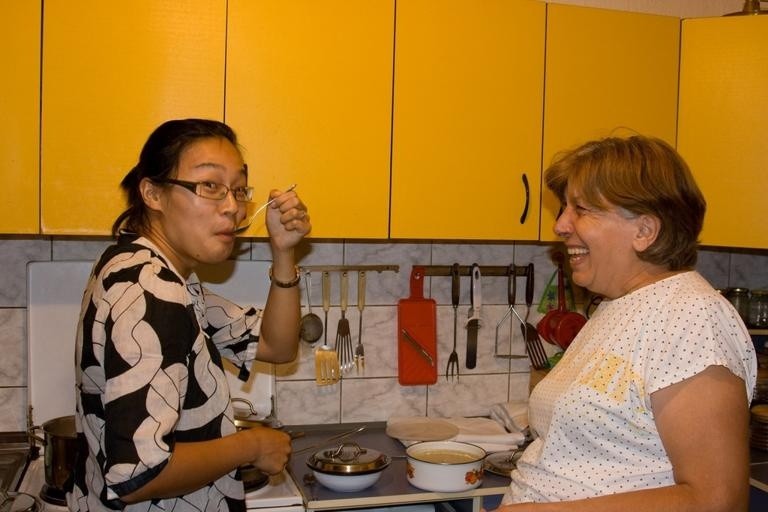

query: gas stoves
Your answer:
[9,454,302,512]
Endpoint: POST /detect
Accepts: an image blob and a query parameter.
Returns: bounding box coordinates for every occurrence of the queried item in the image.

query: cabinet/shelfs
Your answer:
[1,0,768,253]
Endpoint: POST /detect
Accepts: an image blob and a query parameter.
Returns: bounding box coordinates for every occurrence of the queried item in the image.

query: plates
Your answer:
[385,417,462,443]
[484,449,526,478]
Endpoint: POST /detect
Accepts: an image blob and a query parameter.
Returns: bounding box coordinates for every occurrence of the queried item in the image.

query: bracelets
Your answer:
[268,263,302,289]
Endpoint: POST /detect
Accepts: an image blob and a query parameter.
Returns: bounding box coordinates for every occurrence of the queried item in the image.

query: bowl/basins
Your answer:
[314,470,383,494]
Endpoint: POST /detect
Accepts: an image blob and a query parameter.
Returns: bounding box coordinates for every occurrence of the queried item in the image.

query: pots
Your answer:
[403,441,489,494]
[27,415,87,492]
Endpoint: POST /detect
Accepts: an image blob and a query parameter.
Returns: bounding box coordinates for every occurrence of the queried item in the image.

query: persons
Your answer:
[67,118,310,511]
[477,133,758,512]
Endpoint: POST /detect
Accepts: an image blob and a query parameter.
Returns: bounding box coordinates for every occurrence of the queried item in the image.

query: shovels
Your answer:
[315,271,340,386]
[520,263,550,369]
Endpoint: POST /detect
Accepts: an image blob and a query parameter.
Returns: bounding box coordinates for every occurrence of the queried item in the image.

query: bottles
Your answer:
[717,285,768,329]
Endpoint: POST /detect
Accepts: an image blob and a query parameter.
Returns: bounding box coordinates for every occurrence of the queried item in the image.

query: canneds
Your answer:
[715,286,768,329]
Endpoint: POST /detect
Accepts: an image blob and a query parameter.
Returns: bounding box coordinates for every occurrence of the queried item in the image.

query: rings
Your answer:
[299,210,305,220]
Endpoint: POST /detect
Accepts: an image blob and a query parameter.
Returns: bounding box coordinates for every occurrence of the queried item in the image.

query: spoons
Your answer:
[225,184,298,236]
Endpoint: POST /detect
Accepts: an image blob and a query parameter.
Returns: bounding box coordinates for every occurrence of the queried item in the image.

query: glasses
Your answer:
[165,175,254,204]
